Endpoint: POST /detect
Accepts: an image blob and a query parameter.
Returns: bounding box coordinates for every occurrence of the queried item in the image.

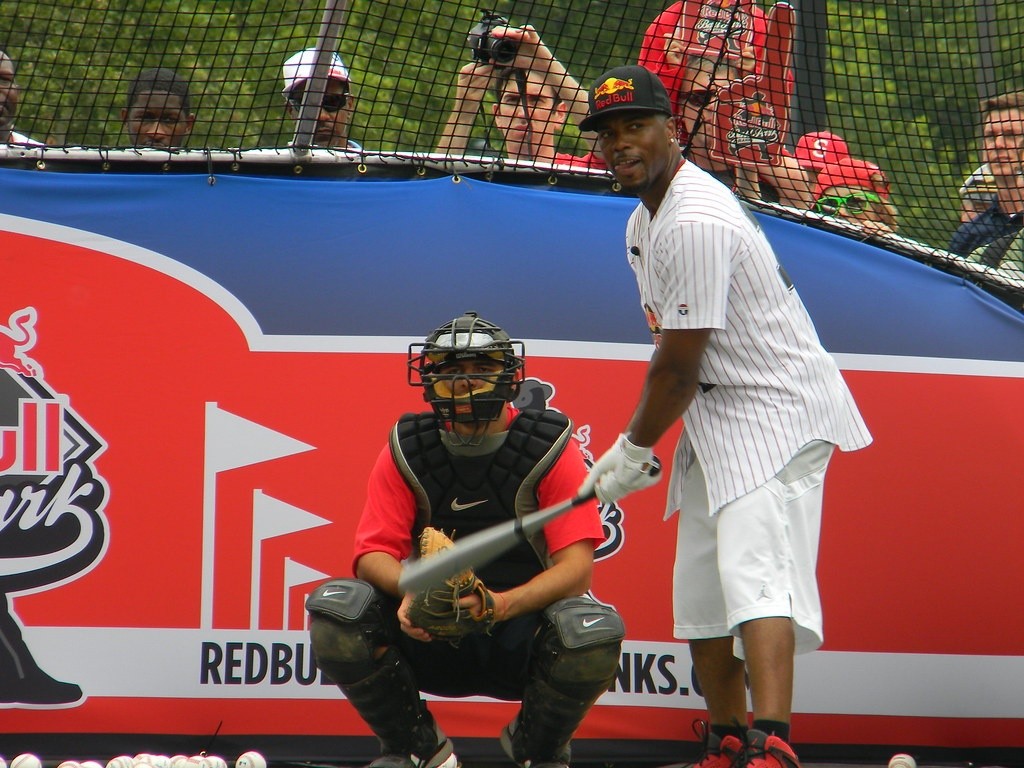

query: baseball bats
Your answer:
[398,457,661,596]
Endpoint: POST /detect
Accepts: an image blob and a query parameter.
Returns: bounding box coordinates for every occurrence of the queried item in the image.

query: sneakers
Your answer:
[736,729,802,768]
[363,698,460,768]
[664,718,744,768]
[500,715,570,768]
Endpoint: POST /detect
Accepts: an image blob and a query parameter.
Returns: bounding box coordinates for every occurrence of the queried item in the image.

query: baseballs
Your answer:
[10,753,42,768]
[888,753,917,768]
[80,761,103,768]
[0,758,7,768]
[236,751,267,768]
[57,761,79,768]
[104,751,227,768]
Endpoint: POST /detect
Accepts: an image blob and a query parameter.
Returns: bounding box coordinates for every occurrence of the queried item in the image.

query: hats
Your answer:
[281,47,352,104]
[958,164,997,204]
[811,158,887,217]
[792,129,850,171]
[579,65,674,135]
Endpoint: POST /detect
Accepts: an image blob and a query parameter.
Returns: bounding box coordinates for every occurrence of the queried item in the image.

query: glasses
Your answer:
[289,90,350,113]
[816,192,883,218]
[678,90,717,107]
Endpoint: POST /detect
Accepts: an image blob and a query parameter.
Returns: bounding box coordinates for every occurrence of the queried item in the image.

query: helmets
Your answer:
[406,310,527,446]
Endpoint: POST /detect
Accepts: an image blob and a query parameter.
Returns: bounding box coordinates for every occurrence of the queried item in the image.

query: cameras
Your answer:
[468,13,518,63]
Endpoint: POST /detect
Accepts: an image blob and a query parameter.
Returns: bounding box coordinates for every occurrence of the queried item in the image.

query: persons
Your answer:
[947,89,1024,254]
[681,58,762,198]
[812,157,899,231]
[278,48,356,150]
[972,141,1024,275]
[1,52,36,144]
[960,167,993,222]
[573,68,877,766]
[433,25,608,169]
[118,73,197,153]
[305,309,627,767]
[796,120,848,182]
[662,2,808,210]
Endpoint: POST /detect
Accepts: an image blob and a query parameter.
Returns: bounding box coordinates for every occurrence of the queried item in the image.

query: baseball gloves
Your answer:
[406,524,496,640]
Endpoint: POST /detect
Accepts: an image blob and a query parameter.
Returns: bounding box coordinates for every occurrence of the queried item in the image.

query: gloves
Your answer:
[578,431,661,503]
